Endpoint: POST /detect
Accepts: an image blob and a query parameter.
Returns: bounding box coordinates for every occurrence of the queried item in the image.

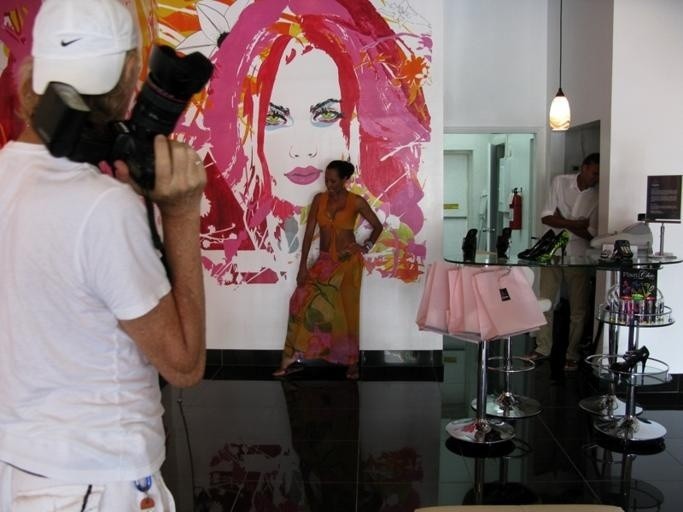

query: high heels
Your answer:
[610,345,649,374]
[517,229,556,261]
[533,229,569,263]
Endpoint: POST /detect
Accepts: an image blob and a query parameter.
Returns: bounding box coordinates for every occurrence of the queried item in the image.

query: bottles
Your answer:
[621,293,656,322]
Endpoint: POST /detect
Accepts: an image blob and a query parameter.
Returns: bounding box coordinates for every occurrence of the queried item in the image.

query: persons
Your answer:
[0,0,209,510]
[517,151,600,372]
[272,160,384,382]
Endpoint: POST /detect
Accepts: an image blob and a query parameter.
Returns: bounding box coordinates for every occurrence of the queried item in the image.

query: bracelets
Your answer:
[364,240,373,250]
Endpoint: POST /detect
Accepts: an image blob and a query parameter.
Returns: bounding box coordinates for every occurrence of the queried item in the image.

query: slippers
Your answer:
[346,370,360,381]
[272,364,305,377]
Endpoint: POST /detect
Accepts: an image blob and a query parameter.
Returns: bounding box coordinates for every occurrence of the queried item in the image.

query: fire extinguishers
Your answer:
[508,188,522,229]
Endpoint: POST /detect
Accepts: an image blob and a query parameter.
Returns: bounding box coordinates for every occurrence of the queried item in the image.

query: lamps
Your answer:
[547,2,572,132]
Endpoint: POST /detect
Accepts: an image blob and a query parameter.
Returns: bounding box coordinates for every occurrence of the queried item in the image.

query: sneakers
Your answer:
[521,351,546,361]
[564,360,580,370]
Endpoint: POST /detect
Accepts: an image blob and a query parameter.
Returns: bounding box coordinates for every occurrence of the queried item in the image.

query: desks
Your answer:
[443,250,682,448]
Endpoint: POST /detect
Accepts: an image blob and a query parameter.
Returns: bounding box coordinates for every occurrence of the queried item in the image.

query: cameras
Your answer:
[32,44,214,190]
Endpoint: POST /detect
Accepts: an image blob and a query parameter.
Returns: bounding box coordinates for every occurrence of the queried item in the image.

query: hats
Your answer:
[31,0,138,97]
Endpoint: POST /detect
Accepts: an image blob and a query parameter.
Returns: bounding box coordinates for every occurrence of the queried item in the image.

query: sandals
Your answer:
[496,227,513,259]
[462,228,478,262]
